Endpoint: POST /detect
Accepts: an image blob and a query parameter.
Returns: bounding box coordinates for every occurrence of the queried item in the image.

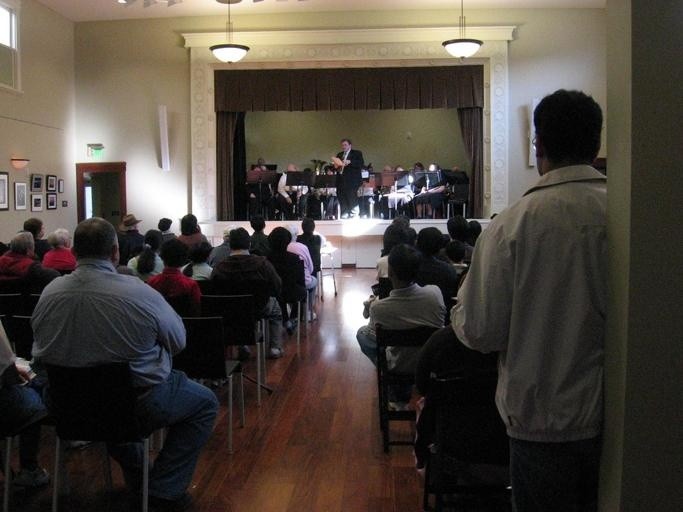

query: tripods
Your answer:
[246,171,275,216]
[382,169,409,210]
[314,175,338,214]
[415,171,440,190]
[445,172,468,217]
[286,171,309,219]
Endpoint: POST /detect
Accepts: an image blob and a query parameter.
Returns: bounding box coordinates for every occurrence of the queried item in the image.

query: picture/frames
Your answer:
[0,172,8,211]
[58,179,64,193]
[46,193,57,210]
[14,181,28,211]
[31,194,44,212]
[31,174,44,192]
[46,175,57,192]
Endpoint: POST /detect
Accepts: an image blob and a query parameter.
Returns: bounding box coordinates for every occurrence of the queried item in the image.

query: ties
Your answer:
[341,151,347,161]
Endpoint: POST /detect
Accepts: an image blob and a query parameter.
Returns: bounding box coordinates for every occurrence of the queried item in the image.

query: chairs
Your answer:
[423,376,475,512]
[269,260,304,347]
[199,295,265,407]
[1,411,48,512]
[171,317,245,456]
[0,266,75,359]
[375,323,440,453]
[36,361,164,512]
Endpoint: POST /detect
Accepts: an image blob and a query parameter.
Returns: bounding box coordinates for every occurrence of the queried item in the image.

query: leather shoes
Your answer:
[270,347,285,357]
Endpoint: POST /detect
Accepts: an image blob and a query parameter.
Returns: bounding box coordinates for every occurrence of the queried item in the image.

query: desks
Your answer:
[316,247,339,304]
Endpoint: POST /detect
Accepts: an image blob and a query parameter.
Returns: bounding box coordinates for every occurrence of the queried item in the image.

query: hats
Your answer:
[118,215,140,232]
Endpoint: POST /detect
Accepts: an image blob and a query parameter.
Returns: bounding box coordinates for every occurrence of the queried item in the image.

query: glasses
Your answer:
[532,136,537,149]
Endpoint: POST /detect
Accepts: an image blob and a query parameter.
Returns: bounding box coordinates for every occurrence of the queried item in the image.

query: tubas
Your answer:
[311,159,322,175]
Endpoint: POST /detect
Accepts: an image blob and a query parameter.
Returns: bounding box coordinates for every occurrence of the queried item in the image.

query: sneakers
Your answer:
[17,469,51,488]
[390,402,409,411]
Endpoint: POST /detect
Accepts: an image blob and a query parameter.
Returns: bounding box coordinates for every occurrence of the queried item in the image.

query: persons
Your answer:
[414,227,459,298]
[118,213,145,267]
[206,228,286,359]
[250,216,269,256]
[1,319,49,493]
[31,217,219,512]
[265,227,306,337]
[412,323,498,479]
[320,165,337,219]
[284,224,317,322]
[127,230,164,283]
[446,242,469,275]
[245,164,275,219]
[440,234,450,250]
[178,214,208,257]
[392,216,416,242]
[296,217,320,274]
[0,231,33,277]
[24,218,52,263]
[37,270,61,292]
[450,90,609,512]
[277,164,308,220]
[415,163,445,218]
[358,162,424,219]
[158,218,177,244]
[447,215,474,265]
[332,139,360,221]
[206,228,232,267]
[180,244,213,282]
[146,238,201,318]
[469,221,482,238]
[363,225,410,319]
[356,245,447,412]
[43,228,76,270]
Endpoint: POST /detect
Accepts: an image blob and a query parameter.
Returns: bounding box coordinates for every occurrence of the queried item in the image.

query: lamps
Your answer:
[209,44,250,65]
[11,158,30,171]
[442,39,483,64]
